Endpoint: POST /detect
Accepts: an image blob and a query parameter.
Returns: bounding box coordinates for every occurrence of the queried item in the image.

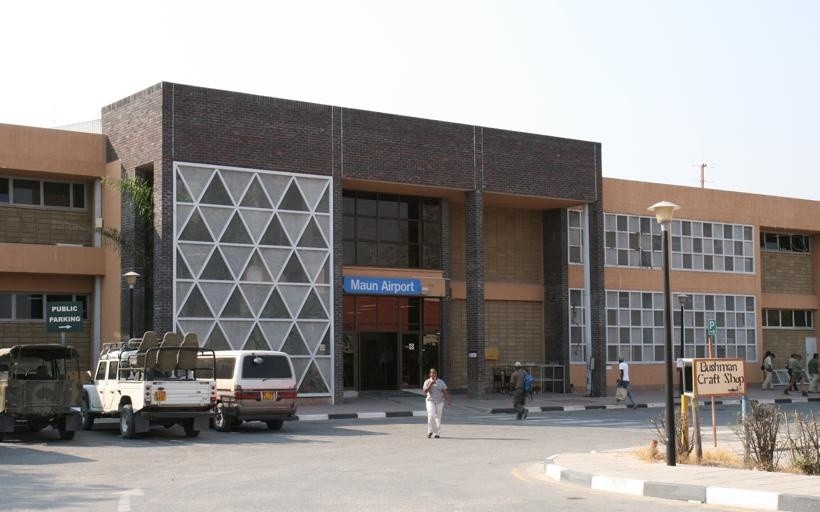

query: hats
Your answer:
[619,356,624,361]
[514,361,521,367]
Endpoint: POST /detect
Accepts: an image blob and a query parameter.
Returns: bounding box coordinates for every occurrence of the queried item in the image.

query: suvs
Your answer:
[0,344,79,442]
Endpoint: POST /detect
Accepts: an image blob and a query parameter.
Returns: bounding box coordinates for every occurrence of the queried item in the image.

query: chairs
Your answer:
[157,332,182,372]
[33,363,48,379]
[177,331,200,370]
[137,330,158,369]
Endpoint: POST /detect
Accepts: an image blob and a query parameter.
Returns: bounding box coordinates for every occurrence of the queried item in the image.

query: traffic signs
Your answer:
[46,303,82,330]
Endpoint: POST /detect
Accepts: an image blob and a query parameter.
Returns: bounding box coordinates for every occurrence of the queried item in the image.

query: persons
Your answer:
[784,352,808,396]
[423,368,452,438]
[616,356,638,410]
[509,361,529,420]
[761,350,776,390]
[808,353,820,393]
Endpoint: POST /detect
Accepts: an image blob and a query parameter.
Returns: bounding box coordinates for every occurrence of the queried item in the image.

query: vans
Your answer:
[199,348,299,430]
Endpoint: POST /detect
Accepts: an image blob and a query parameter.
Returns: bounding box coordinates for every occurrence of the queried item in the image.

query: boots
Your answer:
[802,391,809,396]
[784,388,789,395]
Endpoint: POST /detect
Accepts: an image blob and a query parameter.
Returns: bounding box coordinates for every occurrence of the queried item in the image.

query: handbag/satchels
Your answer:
[616,387,627,400]
[761,361,765,370]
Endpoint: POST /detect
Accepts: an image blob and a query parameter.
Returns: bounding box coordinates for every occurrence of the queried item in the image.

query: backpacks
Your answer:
[522,371,534,392]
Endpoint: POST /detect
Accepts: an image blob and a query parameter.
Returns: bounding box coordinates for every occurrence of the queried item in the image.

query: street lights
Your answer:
[647,200,681,465]
[122,270,142,341]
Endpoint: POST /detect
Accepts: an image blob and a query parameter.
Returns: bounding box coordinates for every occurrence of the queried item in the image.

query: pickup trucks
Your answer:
[79,331,216,439]
